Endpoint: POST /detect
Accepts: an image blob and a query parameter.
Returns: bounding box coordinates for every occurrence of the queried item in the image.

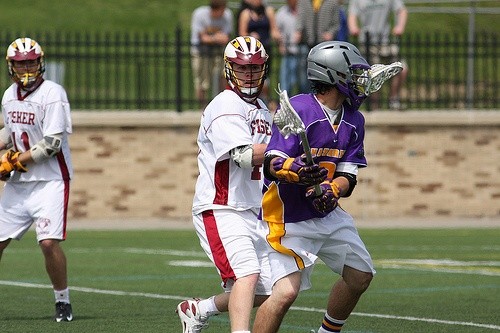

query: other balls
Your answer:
[274,111,287,125]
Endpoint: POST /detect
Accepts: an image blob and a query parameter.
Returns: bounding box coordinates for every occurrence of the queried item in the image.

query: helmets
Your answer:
[225,35,269,103]
[6,37,47,90]
[307,41,371,110]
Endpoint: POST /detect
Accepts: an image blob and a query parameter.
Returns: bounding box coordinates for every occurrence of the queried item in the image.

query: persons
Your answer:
[191,0,409,112]
[0,38,73,322]
[175,36,274,333]
[253,41,377,333]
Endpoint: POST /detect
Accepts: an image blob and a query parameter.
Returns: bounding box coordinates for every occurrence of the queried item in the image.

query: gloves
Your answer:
[0,149,27,182]
[305,180,342,218]
[270,153,329,186]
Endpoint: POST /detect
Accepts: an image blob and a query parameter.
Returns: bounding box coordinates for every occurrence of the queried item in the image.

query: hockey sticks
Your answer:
[358,62,403,96]
[274,84,323,197]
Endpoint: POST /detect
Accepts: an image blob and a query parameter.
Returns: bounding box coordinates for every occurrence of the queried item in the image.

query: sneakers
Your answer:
[177,299,205,333]
[55,298,73,323]
